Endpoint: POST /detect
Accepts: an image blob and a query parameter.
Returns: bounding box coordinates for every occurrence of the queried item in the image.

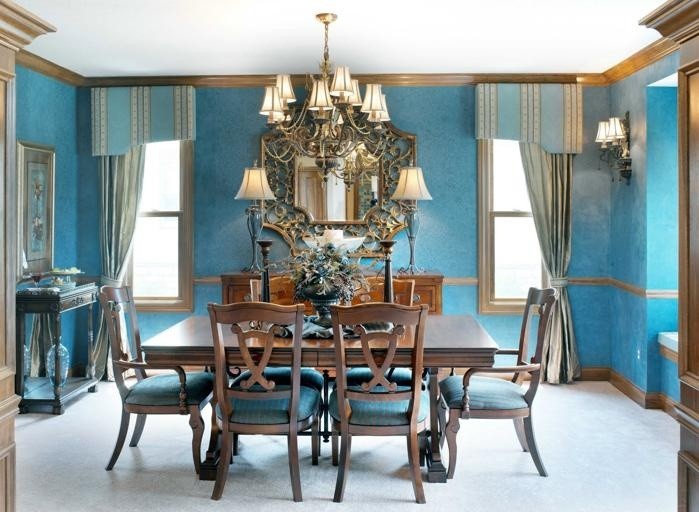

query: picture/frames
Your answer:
[17,140,55,274]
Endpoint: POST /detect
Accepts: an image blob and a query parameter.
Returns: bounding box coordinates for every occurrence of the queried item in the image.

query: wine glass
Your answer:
[29,272,43,289]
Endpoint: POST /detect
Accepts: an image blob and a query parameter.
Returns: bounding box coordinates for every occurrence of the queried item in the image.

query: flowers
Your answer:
[280,243,371,305]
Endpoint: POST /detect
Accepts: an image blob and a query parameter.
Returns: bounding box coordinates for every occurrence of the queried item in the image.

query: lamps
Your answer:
[594,112,632,187]
[232,160,277,274]
[258,14,392,162]
[390,165,433,275]
[330,144,379,192]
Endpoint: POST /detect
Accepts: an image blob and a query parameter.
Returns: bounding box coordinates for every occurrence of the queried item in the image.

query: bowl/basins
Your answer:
[46,282,76,291]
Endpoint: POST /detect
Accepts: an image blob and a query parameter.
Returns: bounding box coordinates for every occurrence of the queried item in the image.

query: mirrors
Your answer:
[256,105,418,258]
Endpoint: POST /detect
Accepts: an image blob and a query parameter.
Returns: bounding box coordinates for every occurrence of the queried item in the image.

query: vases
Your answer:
[311,295,341,320]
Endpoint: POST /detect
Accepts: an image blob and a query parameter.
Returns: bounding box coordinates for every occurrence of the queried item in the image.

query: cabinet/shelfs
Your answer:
[221,270,443,316]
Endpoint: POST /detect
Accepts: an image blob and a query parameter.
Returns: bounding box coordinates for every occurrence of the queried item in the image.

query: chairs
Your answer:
[322,302,434,506]
[101,283,213,480]
[439,286,560,483]
[200,298,326,503]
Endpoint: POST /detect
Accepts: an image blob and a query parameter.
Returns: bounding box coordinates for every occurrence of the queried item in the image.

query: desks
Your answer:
[140,310,499,485]
